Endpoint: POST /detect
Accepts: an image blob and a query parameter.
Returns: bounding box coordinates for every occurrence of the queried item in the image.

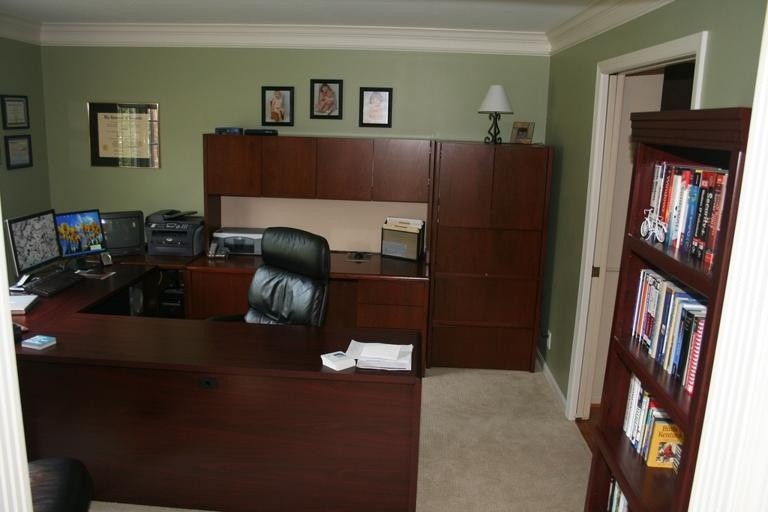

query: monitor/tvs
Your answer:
[54,209,108,273]
[99,211,146,257]
[5,208,66,287]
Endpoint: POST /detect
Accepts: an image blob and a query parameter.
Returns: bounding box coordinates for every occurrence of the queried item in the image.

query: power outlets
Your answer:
[546,329,551,350]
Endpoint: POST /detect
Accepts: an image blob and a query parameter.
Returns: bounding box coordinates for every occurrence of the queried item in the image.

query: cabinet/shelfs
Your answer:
[426,139,554,373]
[585,107,753,512]
[185,134,436,378]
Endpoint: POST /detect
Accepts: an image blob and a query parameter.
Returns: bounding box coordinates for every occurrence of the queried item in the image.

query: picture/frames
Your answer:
[0,95,30,129]
[310,79,343,120]
[87,101,161,169]
[261,85,294,126]
[359,86,393,128]
[510,121,536,144]
[5,135,33,170]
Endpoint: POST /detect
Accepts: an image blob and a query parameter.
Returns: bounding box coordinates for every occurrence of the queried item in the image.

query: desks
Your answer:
[9,265,422,510]
[112,237,205,318]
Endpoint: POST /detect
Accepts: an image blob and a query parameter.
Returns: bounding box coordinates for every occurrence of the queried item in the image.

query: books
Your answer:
[356,343,414,371]
[622,374,684,467]
[632,269,707,395]
[607,477,630,511]
[382,216,424,261]
[648,164,729,272]
[10,295,39,315]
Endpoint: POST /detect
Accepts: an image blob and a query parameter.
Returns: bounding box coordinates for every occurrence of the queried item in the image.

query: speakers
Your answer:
[101,252,113,266]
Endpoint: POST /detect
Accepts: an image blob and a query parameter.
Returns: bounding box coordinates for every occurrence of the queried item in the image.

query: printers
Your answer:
[146,207,204,257]
[213,226,265,256]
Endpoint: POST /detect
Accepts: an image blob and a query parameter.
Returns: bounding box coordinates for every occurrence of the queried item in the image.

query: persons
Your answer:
[272,92,284,120]
[319,84,336,113]
[365,91,386,122]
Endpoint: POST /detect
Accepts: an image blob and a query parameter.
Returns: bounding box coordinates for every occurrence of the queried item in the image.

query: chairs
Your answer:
[205,226,330,327]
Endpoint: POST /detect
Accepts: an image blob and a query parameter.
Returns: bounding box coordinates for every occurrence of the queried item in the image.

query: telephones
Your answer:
[207,242,230,260]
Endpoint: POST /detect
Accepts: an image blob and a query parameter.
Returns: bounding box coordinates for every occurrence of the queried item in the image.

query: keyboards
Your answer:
[30,272,81,298]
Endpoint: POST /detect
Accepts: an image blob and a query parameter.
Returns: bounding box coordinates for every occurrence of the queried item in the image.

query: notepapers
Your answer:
[21,334,56,350]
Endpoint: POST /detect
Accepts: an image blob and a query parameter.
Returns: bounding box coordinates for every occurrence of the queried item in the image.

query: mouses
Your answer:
[86,268,105,274]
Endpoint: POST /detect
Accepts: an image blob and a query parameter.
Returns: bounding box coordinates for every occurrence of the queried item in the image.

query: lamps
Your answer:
[478,85,514,144]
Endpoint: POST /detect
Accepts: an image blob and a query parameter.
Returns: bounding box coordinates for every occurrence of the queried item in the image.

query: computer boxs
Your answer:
[160,288,184,318]
